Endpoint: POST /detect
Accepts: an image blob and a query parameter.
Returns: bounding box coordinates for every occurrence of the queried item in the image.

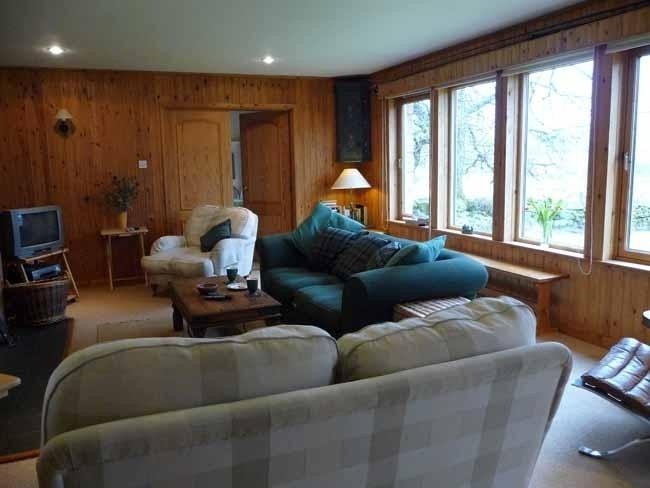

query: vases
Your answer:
[117,211,128,231]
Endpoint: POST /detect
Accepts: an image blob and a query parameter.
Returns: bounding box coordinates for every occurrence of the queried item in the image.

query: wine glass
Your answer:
[247,280,258,297]
[223,266,239,286]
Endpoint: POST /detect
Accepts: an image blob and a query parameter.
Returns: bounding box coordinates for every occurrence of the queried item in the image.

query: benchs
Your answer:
[377,230,569,334]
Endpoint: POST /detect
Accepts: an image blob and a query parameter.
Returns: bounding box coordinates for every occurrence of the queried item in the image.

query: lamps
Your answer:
[330,168,372,206]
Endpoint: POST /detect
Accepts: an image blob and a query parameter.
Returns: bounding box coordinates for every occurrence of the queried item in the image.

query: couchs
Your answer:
[255,202,488,337]
[35,297,574,488]
[139,204,259,297]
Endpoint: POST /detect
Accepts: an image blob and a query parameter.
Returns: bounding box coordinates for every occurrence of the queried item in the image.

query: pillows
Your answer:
[304,227,353,271]
[328,231,391,276]
[199,218,231,253]
[383,233,449,268]
[367,241,401,270]
[284,201,365,266]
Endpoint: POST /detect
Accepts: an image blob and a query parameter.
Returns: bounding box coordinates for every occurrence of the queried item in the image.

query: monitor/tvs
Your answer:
[0,205,64,260]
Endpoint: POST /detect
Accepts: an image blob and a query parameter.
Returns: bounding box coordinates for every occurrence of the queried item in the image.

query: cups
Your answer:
[462,225,473,234]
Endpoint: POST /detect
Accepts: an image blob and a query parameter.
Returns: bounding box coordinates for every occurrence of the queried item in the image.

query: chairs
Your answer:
[572,334,649,459]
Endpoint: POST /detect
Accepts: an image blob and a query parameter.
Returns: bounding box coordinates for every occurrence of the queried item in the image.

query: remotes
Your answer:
[204,294,232,301]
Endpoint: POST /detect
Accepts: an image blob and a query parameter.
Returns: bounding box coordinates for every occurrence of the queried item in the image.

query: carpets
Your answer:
[0,293,75,462]
[93,310,247,345]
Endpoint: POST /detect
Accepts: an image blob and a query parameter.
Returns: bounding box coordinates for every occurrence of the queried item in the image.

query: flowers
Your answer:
[96,174,149,212]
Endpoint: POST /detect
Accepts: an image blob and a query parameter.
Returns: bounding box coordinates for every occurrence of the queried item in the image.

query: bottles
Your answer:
[417,215,426,227]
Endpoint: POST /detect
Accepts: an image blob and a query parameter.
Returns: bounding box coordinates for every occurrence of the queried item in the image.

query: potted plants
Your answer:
[526,194,565,248]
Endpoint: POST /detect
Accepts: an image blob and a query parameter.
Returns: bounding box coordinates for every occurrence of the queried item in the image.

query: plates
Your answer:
[0,373,22,399]
[226,282,248,291]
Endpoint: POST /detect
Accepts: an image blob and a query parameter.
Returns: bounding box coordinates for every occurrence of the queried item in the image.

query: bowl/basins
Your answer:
[195,283,219,294]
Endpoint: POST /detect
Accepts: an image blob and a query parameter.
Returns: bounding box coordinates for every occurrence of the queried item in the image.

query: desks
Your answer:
[101,226,149,289]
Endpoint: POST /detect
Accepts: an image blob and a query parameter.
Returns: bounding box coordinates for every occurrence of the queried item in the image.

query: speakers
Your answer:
[335,75,371,162]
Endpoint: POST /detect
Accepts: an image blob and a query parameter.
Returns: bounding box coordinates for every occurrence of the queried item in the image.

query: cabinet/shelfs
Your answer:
[10,245,82,308]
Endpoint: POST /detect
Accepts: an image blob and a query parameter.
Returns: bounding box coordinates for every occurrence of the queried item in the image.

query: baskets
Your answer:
[1,271,72,328]
